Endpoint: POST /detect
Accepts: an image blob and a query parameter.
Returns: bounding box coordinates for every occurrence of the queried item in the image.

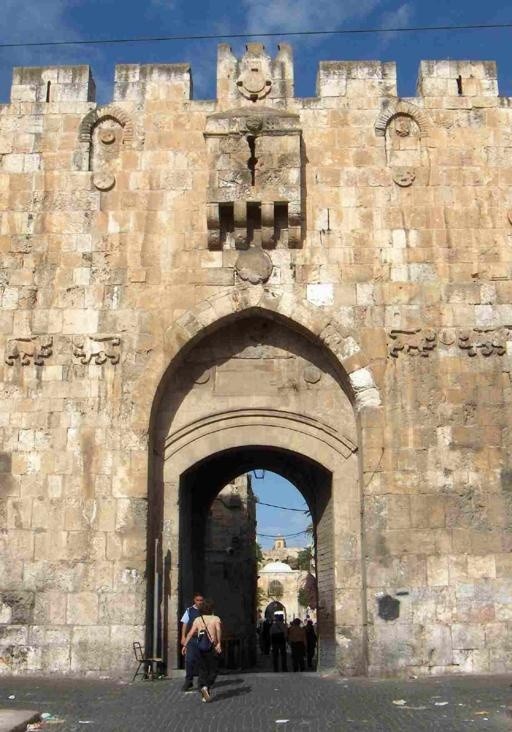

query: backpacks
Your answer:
[197,630,212,651]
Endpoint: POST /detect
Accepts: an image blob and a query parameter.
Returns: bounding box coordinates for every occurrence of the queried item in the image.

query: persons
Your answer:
[257,614,318,673]
[180,592,204,692]
[180,597,222,703]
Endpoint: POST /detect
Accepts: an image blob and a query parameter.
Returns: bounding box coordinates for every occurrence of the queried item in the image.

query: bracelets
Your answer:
[183,646,187,647]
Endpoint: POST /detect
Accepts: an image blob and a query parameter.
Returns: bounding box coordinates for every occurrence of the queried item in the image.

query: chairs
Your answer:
[131,640,163,680]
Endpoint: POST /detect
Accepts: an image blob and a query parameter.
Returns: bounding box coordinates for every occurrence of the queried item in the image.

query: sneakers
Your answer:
[181,681,193,690]
[201,686,210,702]
